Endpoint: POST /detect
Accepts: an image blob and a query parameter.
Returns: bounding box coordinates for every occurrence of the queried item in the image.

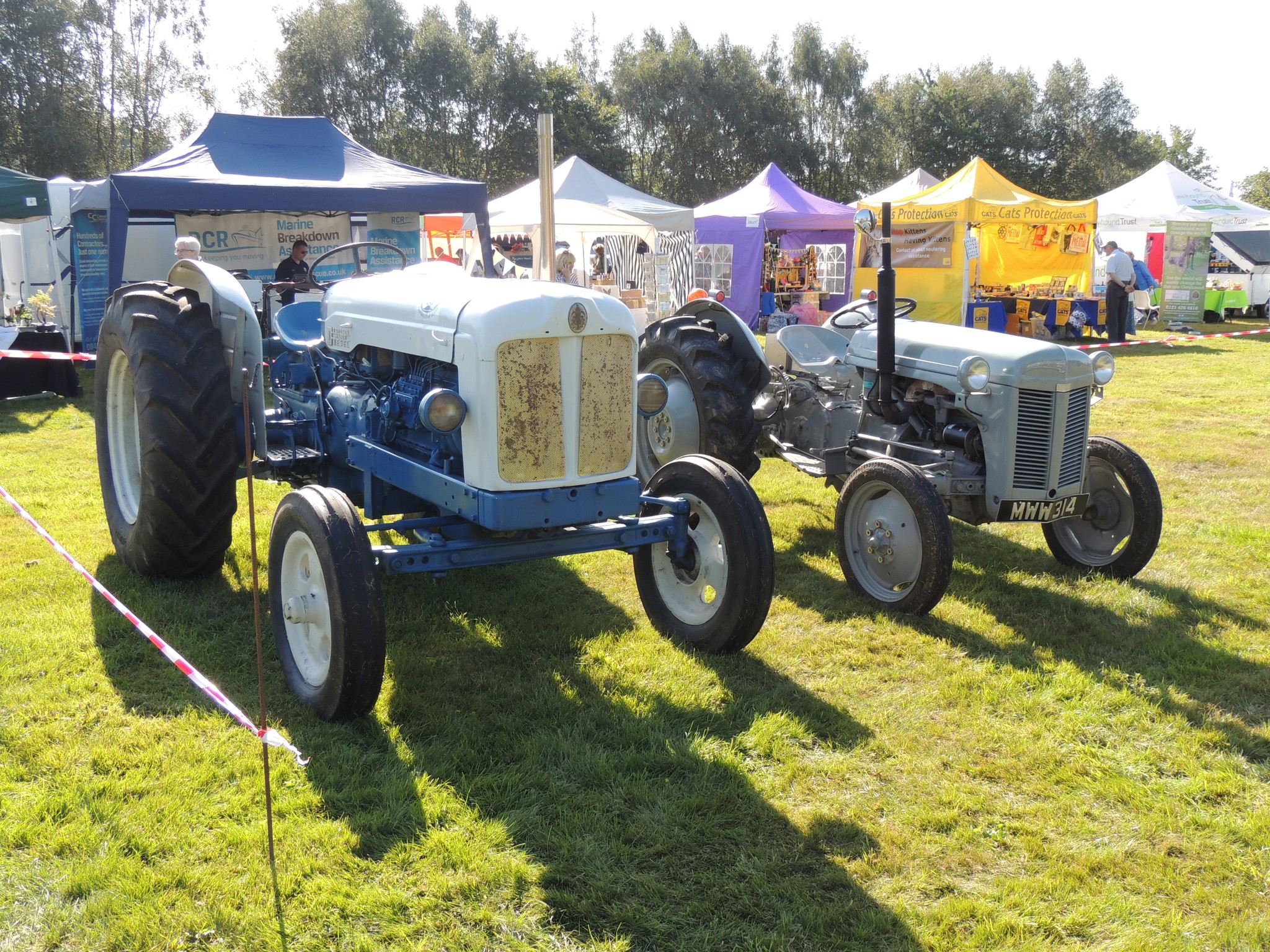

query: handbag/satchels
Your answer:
[607,266,612,274]
[1005,223,1091,255]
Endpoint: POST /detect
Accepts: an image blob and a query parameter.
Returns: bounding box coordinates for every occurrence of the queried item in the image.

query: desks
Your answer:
[966,300,1008,334]
[762,288,829,314]
[1149,288,1246,324]
[976,294,1112,337]
[592,283,620,299]
[0,330,80,399]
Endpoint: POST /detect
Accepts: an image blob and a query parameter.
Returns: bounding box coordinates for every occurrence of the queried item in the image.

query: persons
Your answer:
[591,244,609,273]
[274,240,309,281]
[174,236,204,262]
[1103,241,1158,344]
[556,250,578,285]
[435,247,454,263]
[275,287,310,307]
[456,249,480,277]
[1146,236,1153,253]
[1193,292,1198,299]
[1208,251,1216,273]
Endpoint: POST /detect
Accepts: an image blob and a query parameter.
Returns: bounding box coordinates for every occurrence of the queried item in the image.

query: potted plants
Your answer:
[0,314,16,328]
[27,283,58,331]
[16,307,37,332]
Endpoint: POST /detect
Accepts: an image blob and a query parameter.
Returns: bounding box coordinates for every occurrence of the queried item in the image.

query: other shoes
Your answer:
[1150,317,1158,325]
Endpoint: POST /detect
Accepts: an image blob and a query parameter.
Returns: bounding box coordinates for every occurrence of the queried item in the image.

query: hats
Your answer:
[1099,241,1118,248]
[510,243,522,252]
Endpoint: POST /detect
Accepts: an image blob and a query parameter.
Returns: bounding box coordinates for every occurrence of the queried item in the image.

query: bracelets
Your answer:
[1123,285,1127,289]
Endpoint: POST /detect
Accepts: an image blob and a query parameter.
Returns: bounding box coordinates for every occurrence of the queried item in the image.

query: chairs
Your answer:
[1130,290,1163,331]
[274,299,328,349]
[776,323,852,370]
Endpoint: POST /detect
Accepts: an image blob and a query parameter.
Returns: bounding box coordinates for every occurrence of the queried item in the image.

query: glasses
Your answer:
[1104,246,1111,249]
[298,249,308,255]
[174,249,196,255]
[559,250,572,263]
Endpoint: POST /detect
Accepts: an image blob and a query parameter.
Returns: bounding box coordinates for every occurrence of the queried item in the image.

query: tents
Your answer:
[0,113,1096,367]
[1090,161,1270,286]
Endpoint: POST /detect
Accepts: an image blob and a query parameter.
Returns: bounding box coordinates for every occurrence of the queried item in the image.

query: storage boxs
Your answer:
[791,291,820,302]
[619,281,647,309]
[1005,313,1022,336]
[1029,316,1068,339]
[1020,319,1033,337]
[791,301,820,311]
